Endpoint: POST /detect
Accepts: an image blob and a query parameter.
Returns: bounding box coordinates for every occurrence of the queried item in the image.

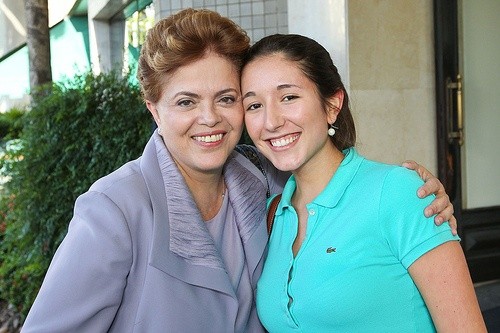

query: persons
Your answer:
[20,6,458,333]
[240,32,488,333]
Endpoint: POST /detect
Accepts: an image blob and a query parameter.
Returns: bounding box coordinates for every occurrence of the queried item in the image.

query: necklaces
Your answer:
[221,173,225,199]
